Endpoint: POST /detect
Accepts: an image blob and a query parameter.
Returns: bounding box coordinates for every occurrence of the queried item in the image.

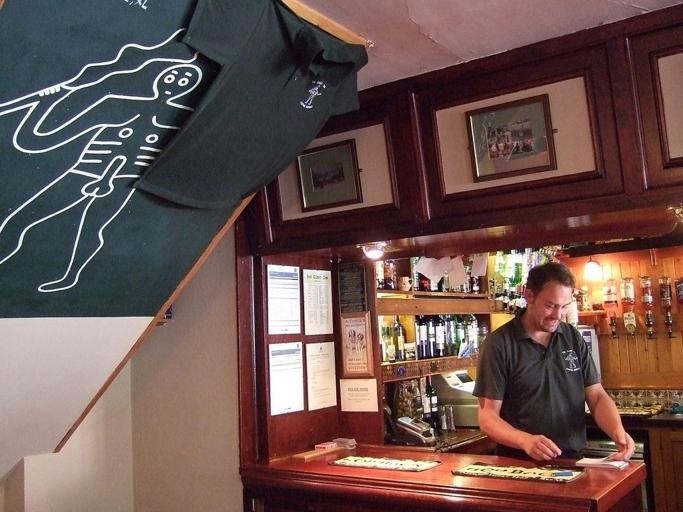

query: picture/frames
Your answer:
[294,138,363,213]
[463,93,558,183]
[336,261,369,314]
[338,309,377,378]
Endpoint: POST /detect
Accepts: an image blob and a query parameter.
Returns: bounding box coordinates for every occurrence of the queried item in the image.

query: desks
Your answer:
[241,442,647,512]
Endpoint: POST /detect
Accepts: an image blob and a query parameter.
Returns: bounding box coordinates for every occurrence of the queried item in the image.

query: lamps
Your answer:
[355,242,387,260]
[582,255,601,279]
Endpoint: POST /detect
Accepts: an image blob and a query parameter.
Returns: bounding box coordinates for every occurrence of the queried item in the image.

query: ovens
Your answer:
[583,451,648,510]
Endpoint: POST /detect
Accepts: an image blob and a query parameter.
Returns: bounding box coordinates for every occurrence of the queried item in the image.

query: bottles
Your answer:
[490,247,533,312]
[619,277,636,306]
[638,276,656,305]
[440,405,447,431]
[393,380,422,420]
[604,280,620,309]
[608,310,620,341]
[373,257,396,291]
[420,374,439,430]
[657,277,673,307]
[622,306,637,339]
[378,314,488,363]
[663,305,673,337]
[447,405,456,431]
[410,257,480,294]
[644,306,657,339]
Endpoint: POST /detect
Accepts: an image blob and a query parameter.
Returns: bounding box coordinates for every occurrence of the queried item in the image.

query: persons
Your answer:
[474,259,637,465]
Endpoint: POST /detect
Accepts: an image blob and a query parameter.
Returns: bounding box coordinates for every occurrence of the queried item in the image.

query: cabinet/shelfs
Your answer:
[649,426,683,511]
[334,258,489,441]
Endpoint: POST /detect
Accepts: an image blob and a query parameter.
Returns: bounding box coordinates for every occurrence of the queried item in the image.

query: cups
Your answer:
[397,276,414,292]
